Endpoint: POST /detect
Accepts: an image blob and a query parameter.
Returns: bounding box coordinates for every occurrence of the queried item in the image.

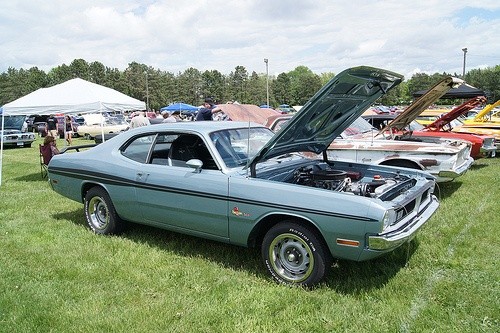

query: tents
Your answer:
[412,77,486,109]
[0,77,146,183]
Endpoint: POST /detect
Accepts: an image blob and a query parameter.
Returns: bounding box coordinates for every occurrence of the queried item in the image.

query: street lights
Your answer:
[144,71,150,112]
[264,58,270,108]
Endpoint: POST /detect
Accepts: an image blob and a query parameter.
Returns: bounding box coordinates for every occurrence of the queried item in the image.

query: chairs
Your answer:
[168,140,211,170]
[39,144,48,179]
[215,136,236,159]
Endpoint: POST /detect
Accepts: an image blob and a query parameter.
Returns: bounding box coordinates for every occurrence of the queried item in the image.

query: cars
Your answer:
[33,121,47,133]
[76,113,129,136]
[217,75,500,186]
[1,115,36,148]
[46,64,439,288]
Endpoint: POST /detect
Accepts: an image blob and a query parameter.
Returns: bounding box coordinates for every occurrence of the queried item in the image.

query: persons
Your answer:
[39,97,219,174]
